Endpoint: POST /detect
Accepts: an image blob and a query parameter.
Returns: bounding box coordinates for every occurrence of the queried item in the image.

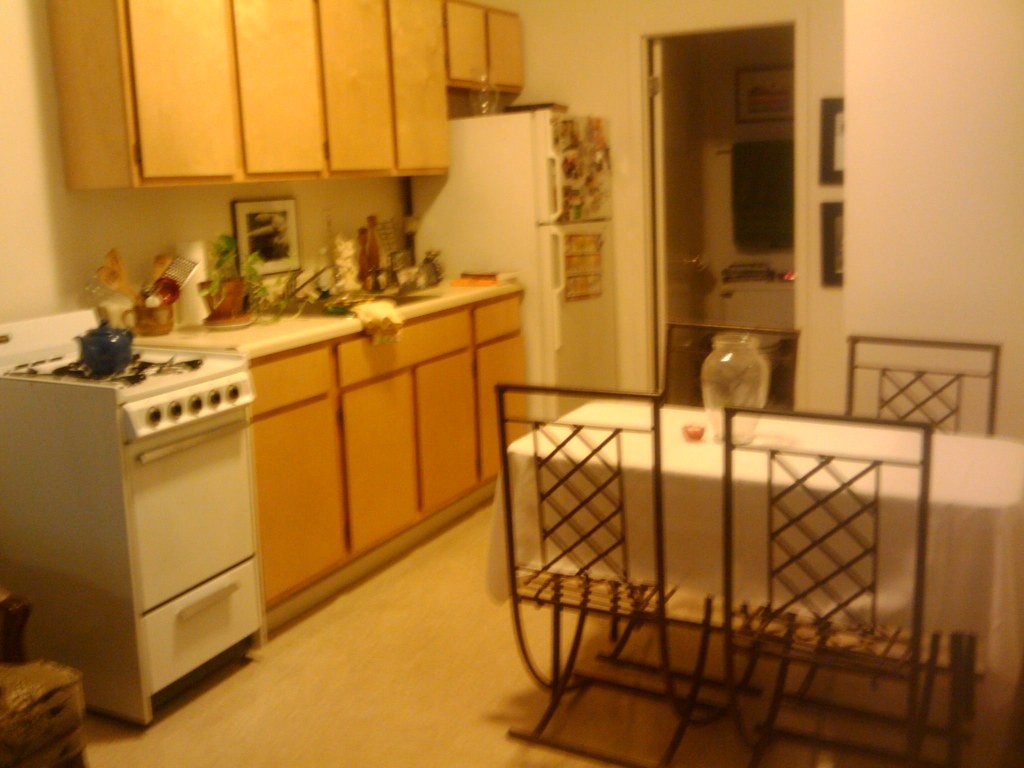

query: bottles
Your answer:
[701,335,769,442]
[358,215,439,291]
[468,71,500,114]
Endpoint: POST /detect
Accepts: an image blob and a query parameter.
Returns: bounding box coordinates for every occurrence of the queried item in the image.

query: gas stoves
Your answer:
[0,309,256,439]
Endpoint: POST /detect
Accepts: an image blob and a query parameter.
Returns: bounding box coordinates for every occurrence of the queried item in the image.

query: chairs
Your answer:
[493,322,1002,768]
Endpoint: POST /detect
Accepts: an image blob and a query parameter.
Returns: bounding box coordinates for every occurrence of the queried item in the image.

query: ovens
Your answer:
[0,392,264,725]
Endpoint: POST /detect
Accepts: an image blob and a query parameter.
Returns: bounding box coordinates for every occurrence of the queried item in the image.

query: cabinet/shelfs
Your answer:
[45,0,523,195]
[249,290,530,615]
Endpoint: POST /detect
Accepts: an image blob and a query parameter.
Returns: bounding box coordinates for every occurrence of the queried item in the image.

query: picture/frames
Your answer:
[819,201,843,287]
[230,195,302,280]
[732,64,793,124]
[819,98,843,186]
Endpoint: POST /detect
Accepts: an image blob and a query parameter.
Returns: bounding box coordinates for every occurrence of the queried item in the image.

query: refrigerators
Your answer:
[410,110,619,422]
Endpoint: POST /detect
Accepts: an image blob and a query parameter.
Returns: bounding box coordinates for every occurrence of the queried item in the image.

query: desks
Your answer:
[488,398,1024,722]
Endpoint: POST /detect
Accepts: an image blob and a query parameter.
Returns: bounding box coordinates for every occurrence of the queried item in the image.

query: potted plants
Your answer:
[198,233,267,316]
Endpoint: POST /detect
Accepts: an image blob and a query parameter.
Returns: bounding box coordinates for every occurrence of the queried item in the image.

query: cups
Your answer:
[686,416,707,444]
[94,301,175,336]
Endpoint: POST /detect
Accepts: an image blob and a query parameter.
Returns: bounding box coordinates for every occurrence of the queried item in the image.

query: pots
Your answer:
[72,320,135,375]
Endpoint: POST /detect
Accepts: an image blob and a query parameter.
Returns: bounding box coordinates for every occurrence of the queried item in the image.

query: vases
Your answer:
[701,333,771,446]
